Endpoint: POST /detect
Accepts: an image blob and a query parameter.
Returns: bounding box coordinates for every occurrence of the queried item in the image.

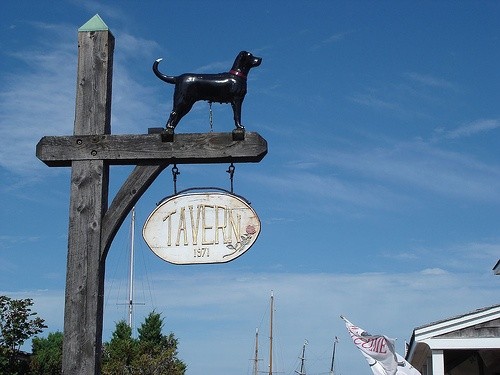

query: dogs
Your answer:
[153,51,262,129]
